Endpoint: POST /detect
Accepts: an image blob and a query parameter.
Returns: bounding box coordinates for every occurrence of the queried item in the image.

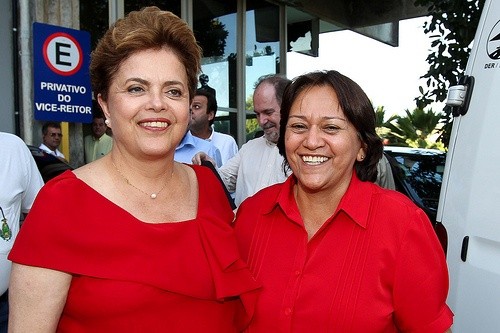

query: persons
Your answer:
[234,70,454,333]
[0,131,45,333]
[7,6,261,333]
[38,121,69,165]
[85,115,113,164]
[173,74,396,223]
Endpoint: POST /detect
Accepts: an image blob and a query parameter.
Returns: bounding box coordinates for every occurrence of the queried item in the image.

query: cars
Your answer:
[380,146,449,234]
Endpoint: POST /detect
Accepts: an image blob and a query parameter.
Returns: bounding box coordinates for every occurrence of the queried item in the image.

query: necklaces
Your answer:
[111,151,174,199]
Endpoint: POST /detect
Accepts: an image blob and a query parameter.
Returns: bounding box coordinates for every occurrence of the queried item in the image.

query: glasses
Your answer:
[47,133,63,137]
